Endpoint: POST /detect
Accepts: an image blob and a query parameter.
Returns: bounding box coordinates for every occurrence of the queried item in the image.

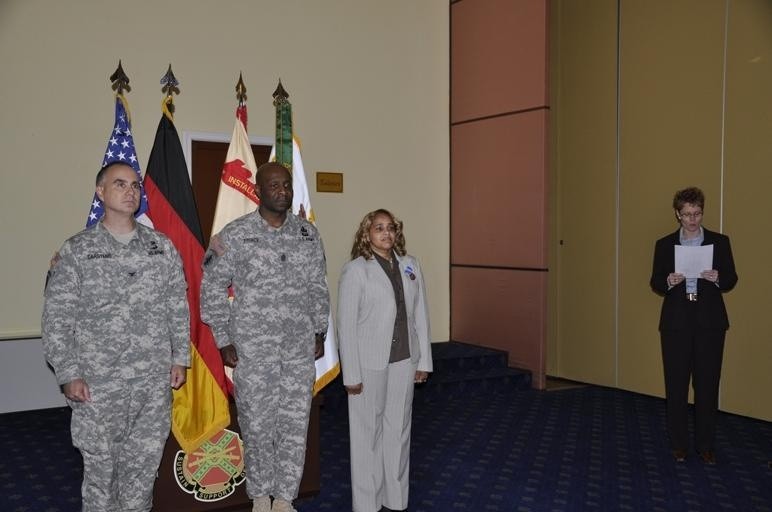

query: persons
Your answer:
[647,185,739,467]
[198,161,332,512]
[335,207,434,512]
[40,161,192,512]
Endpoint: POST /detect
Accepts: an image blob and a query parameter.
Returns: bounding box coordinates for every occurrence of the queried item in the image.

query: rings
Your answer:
[710,273,712,276]
[674,279,676,282]
[710,275,712,279]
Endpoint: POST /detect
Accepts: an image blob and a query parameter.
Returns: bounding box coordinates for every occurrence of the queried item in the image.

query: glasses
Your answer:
[679,210,703,218]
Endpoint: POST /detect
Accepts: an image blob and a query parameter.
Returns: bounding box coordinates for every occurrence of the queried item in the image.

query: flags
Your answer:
[206,92,263,399]
[134,89,233,457]
[269,93,344,405]
[84,89,152,234]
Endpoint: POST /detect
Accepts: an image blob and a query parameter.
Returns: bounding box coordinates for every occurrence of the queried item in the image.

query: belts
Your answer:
[685,292,697,301]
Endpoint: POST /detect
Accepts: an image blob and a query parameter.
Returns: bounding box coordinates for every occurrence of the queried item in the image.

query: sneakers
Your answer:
[272,497,298,512]
[252,494,272,512]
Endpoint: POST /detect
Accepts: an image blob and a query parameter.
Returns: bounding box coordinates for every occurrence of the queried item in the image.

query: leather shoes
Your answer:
[699,452,718,468]
[670,448,689,464]
